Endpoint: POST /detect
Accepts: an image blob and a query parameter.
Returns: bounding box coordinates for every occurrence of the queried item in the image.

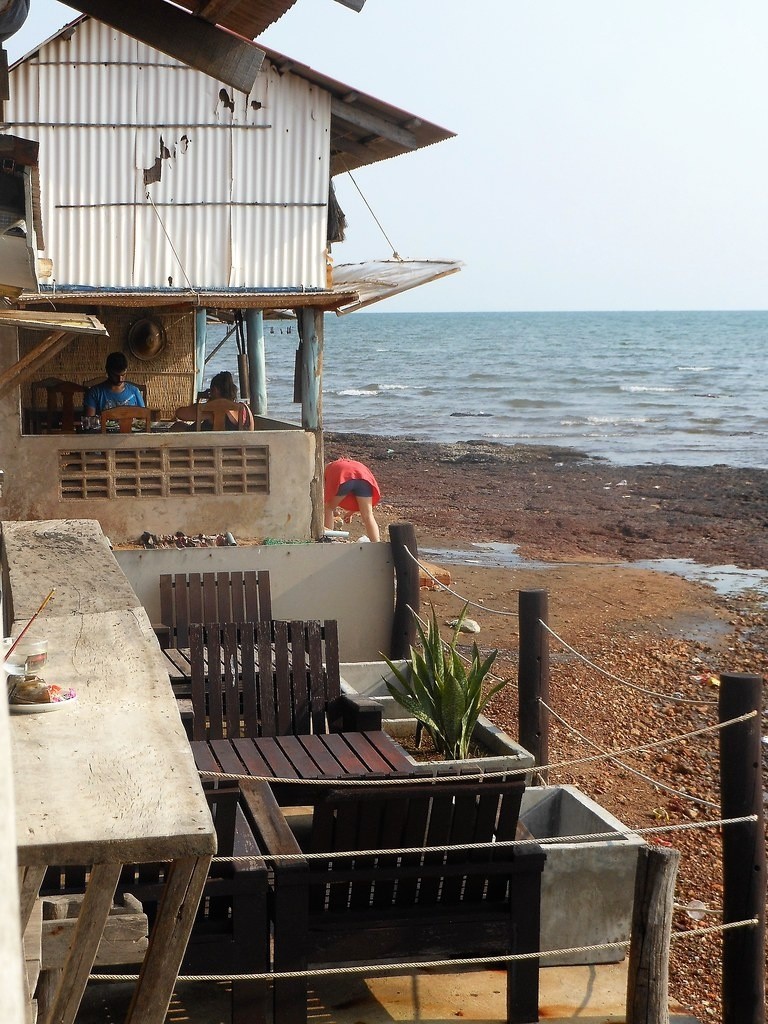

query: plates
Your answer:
[105,420,175,431]
[9,693,77,713]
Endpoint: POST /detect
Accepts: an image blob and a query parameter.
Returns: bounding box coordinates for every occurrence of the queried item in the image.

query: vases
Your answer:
[519,784,649,966]
[321,658,418,719]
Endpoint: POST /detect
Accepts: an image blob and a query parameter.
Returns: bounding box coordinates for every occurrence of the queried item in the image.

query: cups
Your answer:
[0,636,49,676]
[80,415,100,429]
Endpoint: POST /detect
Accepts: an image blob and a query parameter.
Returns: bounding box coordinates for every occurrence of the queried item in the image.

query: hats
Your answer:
[127,316,167,361]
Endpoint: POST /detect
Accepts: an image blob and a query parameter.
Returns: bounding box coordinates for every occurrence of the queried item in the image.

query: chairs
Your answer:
[31,377,89,435]
[128,381,149,406]
[38,571,547,1024]
[196,399,245,430]
[101,405,151,433]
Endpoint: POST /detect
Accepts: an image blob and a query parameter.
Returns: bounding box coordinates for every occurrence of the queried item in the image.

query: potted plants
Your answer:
[376,594,536,785]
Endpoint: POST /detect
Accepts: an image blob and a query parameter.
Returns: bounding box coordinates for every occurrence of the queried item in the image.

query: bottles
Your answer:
[226,532,237,546]
[215,532,228,547]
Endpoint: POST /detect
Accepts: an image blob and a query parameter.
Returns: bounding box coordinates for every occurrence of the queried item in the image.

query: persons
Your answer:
[324,459,380,542]
[176,372,254,430]
[84,352,146,421]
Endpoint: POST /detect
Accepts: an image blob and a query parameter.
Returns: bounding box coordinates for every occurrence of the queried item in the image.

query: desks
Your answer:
[188,730,413,809]
[4,605,216,1024]
[162,641,327,712]
[58,421,194,433]
[25,406,161,436]
